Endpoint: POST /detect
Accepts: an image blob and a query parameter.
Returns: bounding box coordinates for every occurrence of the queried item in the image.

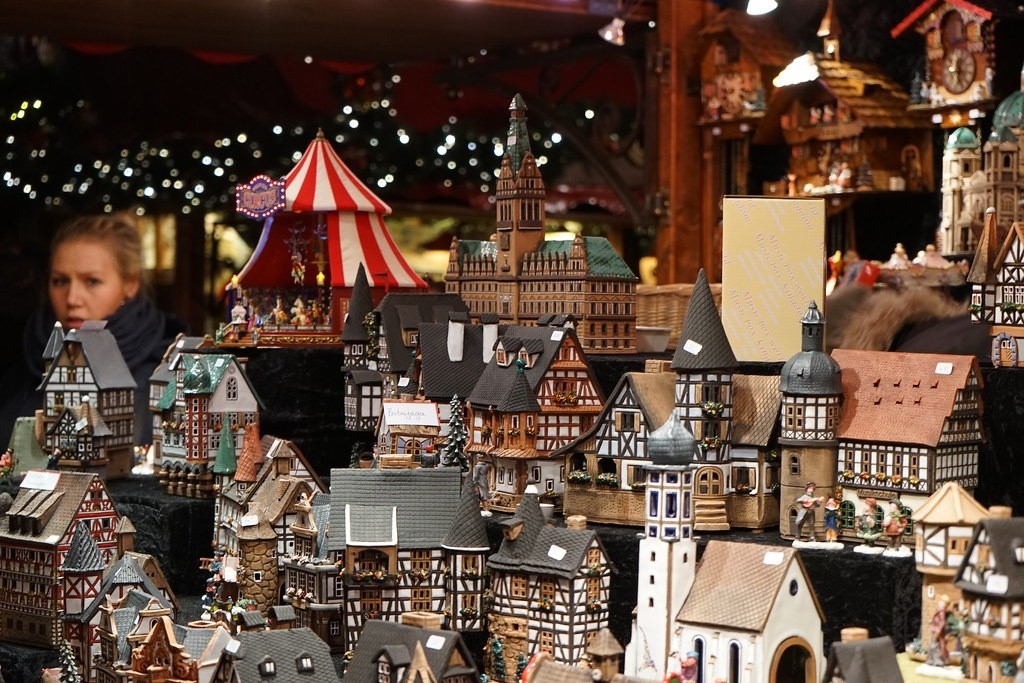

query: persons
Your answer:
[1,217,175,450]
[290,250,306,286]
[474,454,490,511]
[795,482,822,542]
[681,652,699,683]
[925,595,952,667]
[370,445,378,469]
[822,491,843,543]
[880,499,908,551]
[883,242,954,270]
[856,497,881,546]
[47,448,61,470]
[949,601,970,676]
[0,448,14,475]
[132,446,142,466]
[822,284,1024,518]
[208,550,224,578]
[228,274,328,326]
[205,578,217,611]
